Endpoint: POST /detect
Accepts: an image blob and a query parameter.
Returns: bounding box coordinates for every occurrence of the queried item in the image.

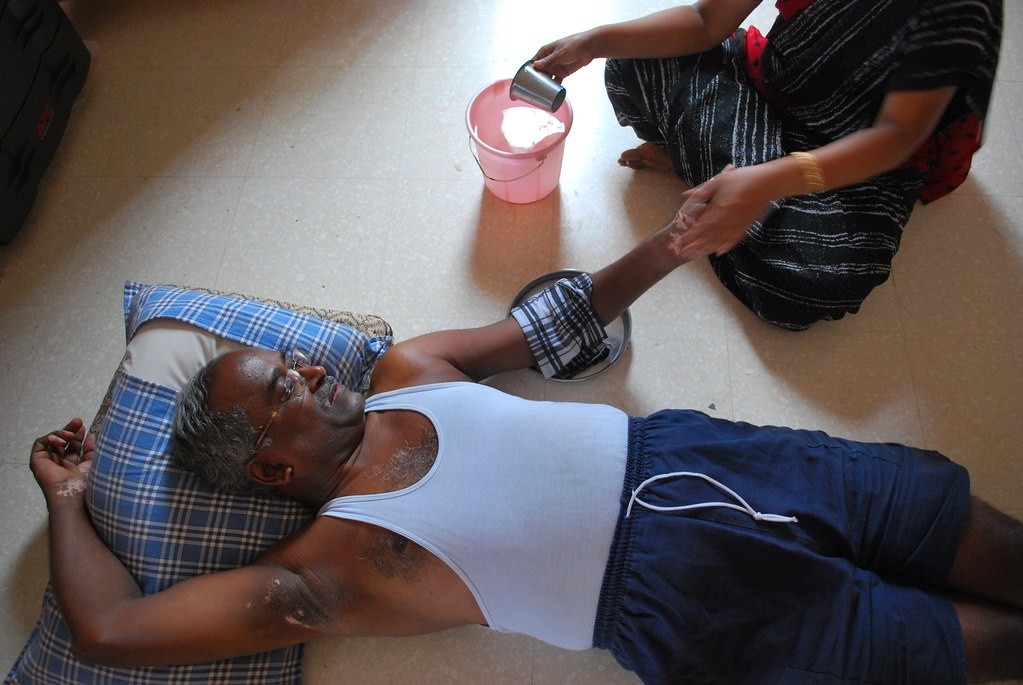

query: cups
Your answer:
[510,60,566,114]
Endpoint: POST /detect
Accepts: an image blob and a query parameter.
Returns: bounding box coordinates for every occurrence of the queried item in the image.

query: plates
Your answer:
[506,269,633,383]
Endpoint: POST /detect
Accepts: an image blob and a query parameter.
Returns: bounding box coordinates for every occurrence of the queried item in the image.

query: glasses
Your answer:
[243,348,311,469]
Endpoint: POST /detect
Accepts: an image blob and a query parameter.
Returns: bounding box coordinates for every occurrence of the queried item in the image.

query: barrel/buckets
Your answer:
[465,77,573,204]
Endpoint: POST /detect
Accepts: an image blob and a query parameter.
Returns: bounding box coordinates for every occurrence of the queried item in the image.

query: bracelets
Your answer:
[792,152,824,193]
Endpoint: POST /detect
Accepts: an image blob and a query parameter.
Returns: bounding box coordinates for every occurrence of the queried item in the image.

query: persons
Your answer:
[28,166,1023,685]
[532,0,1004,332]
